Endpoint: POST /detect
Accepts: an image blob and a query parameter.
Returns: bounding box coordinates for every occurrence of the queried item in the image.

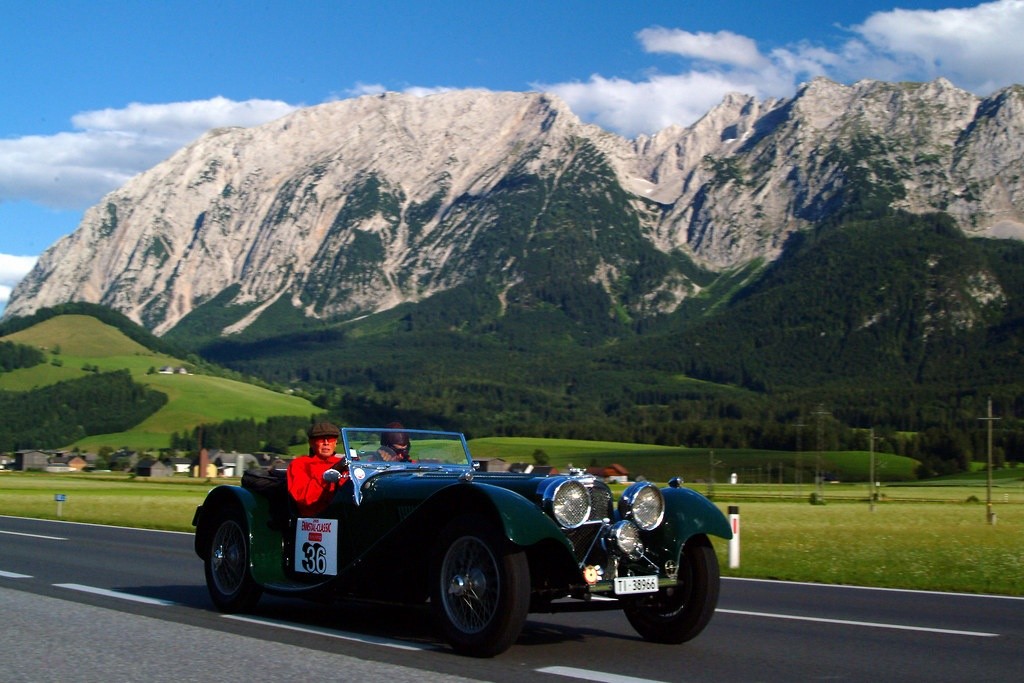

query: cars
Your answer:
[191,426,734,659]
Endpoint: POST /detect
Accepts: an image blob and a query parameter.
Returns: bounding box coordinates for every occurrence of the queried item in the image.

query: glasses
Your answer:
[387,444,408,455]
[313,436,337,444]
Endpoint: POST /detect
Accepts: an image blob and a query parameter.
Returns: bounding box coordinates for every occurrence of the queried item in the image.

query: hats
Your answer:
[307,422,339,439]
[380,423,409,446]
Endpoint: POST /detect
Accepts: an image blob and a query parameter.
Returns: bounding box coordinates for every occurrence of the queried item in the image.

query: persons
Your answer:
[287,423,391,517]
[369,423,417,463]
